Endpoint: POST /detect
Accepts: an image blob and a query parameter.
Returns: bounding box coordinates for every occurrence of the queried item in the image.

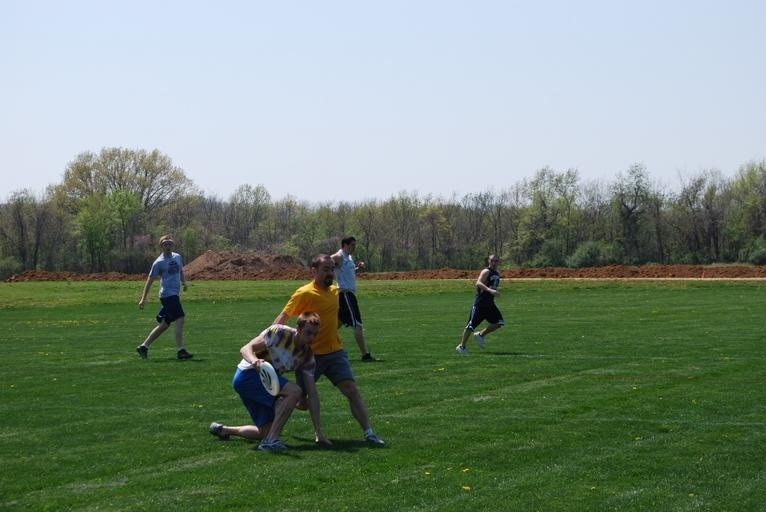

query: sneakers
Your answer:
[136,344,148,359]
[176,349,193,359]
[360,352,376,361]
[209,422,230,441]
[365,435,386,445]
[455,343,467,352]
[258,440,288,451]
[473,331,487,349]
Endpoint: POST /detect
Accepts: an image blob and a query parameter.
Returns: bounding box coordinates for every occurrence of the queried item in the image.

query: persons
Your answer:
[331,237,378,360]
[456,253,504,352]
[273,253,384,445]
[209,311,332,449]
[136,236,193,359]
